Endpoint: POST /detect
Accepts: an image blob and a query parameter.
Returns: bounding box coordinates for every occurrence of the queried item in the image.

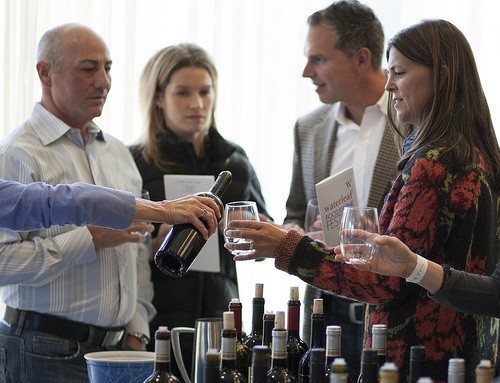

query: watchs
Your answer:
[127,330,148,344]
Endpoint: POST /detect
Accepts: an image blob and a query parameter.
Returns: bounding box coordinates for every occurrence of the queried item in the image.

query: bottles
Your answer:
[154,171,232,278]
[141,283,494,383]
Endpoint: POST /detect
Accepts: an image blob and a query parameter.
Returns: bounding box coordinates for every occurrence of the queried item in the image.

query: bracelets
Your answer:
[406,254,428,284]
[437,264,451,290]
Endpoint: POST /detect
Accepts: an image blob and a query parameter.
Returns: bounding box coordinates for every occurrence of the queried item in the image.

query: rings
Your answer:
[248,242,254,251]
[200,209,208,218]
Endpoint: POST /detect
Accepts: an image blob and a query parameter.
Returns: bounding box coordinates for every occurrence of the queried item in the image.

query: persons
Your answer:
[0,1,500,382]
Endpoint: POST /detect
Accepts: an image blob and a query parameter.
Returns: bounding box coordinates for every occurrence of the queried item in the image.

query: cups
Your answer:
[127,190,152,239]
[303,199,325,244]
[339,206,379,265]
[83,350,154,383]
[224,201,261,255]
